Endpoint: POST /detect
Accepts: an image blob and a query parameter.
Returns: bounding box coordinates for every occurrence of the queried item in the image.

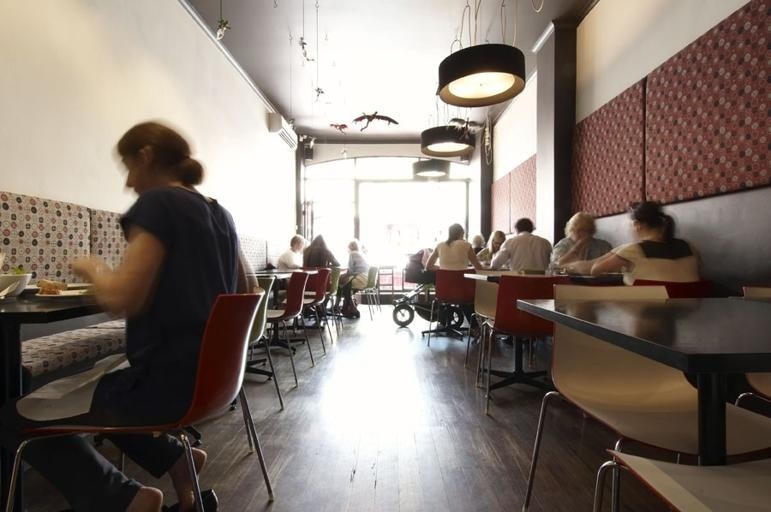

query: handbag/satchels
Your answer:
[342,301,359,317]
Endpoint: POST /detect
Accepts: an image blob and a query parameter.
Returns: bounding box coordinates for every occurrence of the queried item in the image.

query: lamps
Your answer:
[413,1,525,179]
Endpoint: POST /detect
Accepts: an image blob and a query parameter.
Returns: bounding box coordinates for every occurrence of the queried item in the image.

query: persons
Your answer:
[476,230,506,261]
[548,211,613,276]
[327,239,368,318]
[490,218,552,350]
[592,201,705,286]
[426,223,484,331]
[17,122,250,511]
[304,234,340,314]
[277,234,305,267]
[470,235,486,256]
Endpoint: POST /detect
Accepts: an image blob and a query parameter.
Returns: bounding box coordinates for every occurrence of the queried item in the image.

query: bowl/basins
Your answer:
[0,273,32,300]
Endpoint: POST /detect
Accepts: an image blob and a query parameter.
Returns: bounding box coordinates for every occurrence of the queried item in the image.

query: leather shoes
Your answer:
[162,489,219,511]
[501,336,513,343]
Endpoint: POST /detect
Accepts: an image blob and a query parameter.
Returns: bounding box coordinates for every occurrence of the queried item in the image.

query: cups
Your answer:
[549,248,560,274]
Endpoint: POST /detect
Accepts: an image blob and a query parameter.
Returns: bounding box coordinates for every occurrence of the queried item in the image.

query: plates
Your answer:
[24,283,94,290]
[34,289,88,298]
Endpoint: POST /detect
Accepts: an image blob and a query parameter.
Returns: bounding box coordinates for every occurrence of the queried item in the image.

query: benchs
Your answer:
[0,192,128,388]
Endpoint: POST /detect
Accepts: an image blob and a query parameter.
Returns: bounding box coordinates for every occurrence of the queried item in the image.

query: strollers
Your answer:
[392,248,463,329]
